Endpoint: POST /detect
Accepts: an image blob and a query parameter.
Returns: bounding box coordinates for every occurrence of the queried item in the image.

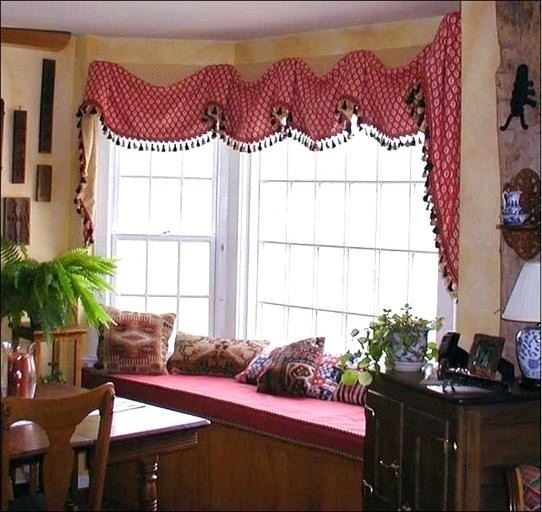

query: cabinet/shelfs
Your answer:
[363,376,541,512]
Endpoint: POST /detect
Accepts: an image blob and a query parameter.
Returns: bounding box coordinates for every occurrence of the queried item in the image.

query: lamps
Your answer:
[500,262,542,380]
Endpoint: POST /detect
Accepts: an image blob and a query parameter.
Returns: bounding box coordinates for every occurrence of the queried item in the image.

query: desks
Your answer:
[7,319,88,388]
[8,386,212,511]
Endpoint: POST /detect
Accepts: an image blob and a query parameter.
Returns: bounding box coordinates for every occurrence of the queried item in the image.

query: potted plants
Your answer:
[338,302,448,392]
[0,234,119,352]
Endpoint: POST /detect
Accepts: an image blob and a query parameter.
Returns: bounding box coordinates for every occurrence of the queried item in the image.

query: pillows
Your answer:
[96,302,373,416]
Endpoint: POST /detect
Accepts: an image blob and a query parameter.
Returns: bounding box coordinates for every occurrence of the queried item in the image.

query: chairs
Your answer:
[0,381,115,512]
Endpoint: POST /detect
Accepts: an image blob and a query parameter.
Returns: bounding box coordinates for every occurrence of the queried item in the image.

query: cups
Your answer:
[1,339,38,399]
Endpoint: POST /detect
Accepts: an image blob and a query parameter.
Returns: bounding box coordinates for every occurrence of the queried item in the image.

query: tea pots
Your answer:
[499,187,531,226]
